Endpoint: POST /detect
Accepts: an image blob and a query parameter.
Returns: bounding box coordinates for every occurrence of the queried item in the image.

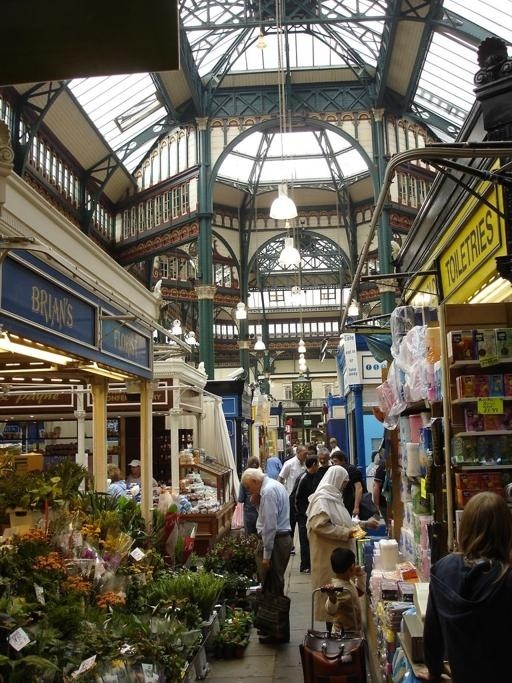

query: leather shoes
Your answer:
[259,635,291,644]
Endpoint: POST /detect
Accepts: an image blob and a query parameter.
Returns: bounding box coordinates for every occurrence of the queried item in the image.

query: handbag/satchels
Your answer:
[252,589,291,633]
[349,493,377,521]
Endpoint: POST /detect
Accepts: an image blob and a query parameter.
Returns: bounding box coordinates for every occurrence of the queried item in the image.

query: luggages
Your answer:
[299,586,367,682]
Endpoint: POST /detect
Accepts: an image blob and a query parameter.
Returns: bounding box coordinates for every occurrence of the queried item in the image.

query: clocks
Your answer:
[292,381,311,401]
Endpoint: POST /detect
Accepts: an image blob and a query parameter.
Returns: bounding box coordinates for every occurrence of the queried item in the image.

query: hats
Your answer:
[128,459,140,468]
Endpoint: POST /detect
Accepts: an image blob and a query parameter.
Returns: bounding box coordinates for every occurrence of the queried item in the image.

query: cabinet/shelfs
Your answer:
[365,534,436,681]
[438,311,512,544]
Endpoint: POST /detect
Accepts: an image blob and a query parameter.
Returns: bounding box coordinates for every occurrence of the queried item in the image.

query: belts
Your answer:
[257,532,290,539]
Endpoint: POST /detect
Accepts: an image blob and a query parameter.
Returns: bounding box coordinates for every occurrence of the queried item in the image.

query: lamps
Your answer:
[236,301,246,320]
[279,237,300,264]
[269,187,298,220]
[254,329,266,351]
[172,318,197,345]
[298,337,307,354]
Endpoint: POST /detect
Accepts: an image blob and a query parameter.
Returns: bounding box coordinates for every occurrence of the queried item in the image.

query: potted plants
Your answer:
[1,455,265,681]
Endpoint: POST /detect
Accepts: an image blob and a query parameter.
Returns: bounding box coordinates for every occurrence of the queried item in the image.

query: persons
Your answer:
[306,464,378,638]
[86,458,158,508]
[242,468,293,645]
[424,492,510,681]
[319,547,365,639]
[237,437,390,582]
